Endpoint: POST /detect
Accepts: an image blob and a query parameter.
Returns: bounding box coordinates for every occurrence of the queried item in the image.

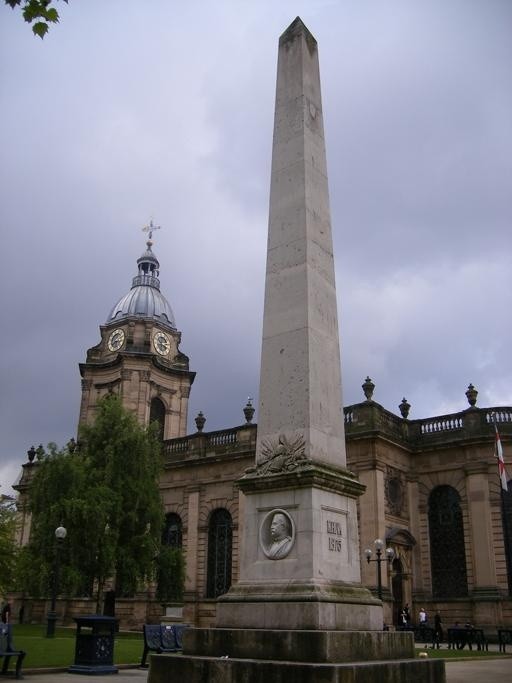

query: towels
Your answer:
[67,615,119,674]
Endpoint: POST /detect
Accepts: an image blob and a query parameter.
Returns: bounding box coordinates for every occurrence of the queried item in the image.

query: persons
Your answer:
[452,620,460,650]
[267,513,293,557]
[19,604,25,623]
[399,602,412,628]
[417,607,429,636]
[458,621,473,650]
[434,609,446,641]
[496,624,508,652]
[1,596,17,651]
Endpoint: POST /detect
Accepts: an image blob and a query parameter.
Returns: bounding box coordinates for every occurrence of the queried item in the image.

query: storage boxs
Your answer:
[0,619,28,681]
[141,623,196,667]
[397,628,512,652]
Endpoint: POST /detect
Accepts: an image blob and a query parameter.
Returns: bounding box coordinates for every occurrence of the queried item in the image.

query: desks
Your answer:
[364,540,394,601]
[45,525,68,637]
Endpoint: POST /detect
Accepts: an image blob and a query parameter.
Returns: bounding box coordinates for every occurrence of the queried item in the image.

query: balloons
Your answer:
[107,328,125,352]
[153,331,171,356]
